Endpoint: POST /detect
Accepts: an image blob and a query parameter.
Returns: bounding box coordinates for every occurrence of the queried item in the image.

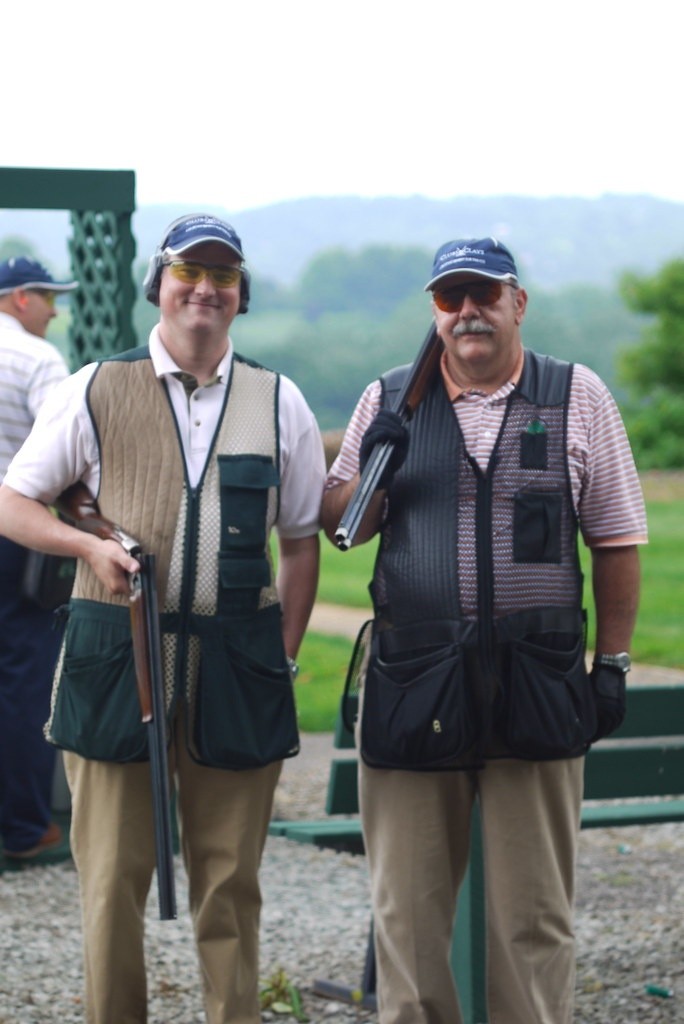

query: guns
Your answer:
[52,480,186,923]
[334,314,445,554]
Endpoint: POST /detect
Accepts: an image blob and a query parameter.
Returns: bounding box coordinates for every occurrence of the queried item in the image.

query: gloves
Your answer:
[586,662,626,744]
[359,410,410,492]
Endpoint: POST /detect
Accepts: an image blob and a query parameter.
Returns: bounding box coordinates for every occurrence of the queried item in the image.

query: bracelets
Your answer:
[287,656,299,679]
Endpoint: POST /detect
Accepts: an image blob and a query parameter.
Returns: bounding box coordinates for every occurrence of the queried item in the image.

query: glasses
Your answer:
[26,290,55,307]
[432,277,503,313]
[166,261,247,288]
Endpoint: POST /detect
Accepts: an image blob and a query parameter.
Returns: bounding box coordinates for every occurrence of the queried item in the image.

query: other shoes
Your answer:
[0,827,73,871]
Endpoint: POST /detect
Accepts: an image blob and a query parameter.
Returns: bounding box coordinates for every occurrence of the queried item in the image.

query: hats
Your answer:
[0,256,80,296]
[162,216,246,262]
[424,237,519,291]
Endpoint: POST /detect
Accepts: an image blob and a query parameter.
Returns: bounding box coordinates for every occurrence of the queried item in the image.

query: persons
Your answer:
[0,255,72,861]
[1,212,328,1024]
[320,235,649,1024]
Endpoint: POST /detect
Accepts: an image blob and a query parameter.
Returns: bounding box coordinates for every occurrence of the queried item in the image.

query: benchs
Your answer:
[268,683,684,1024]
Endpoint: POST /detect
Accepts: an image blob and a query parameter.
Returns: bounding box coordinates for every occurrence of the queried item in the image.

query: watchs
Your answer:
[592,651,631,672]
